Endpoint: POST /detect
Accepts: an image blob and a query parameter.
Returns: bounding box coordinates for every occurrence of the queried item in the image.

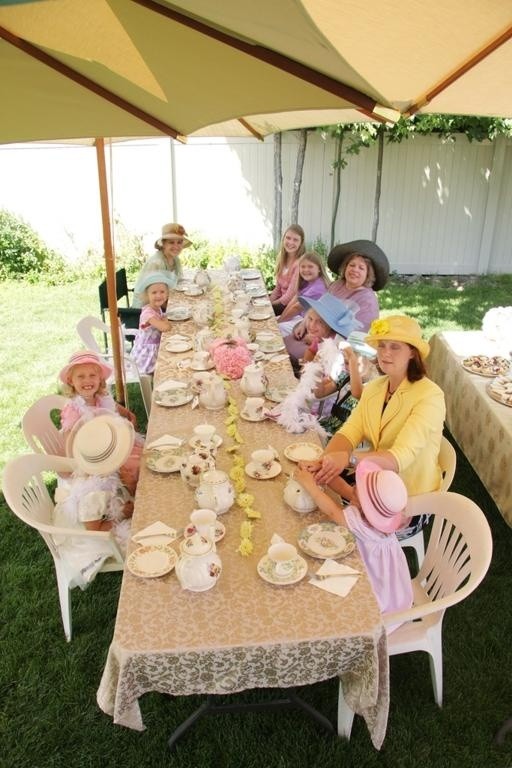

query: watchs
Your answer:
[348,448,357,468]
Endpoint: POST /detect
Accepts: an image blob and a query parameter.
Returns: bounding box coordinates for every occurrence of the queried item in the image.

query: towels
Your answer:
[256,332,276,341]
[265,353,290,363]
[146,434,184,451]
[132,521,183,546]
[308,558,360,597]
[166,334,190,342]
[155,380,186,390]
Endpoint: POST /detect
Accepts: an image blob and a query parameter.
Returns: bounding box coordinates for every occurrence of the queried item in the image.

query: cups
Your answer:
[193,424,217,444]
[192,370,209,393]
[192,350,210,372]
[267,541,298,582]
[188,507,218,551]
[250,449,275,476]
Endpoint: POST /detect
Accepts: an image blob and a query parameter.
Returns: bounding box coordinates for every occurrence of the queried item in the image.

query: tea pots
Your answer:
[193,327,214,350]
[192,269,211,286]
[192,300,213,326]
[194,457,238,518]
[234,317,256,343]
[282,475,325,516]
[174,531,224,596]
[238,358,268,399]
[222,254,240,271]
[197,373,229,413]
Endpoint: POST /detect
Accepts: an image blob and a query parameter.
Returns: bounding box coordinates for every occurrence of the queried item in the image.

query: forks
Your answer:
[310,570,363,582]
[133,529,185,543]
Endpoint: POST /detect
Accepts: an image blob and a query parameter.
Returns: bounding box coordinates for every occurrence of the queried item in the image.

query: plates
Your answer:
[247,312,272,321]
[188,356,216,371]
[189,433,223,450]
[485,376,512,407]
[283,442,325,463]
[144,447,190,477]
[263,381,299,403]
[152,385,194,407]
[242,272,261,280]
[461,352,512,376]
[183,514,227,544]
[164,340,193,353]
[246,283,267,298]
[259,340,287,353]
[166,305,192,322]
[296,520,355,561]
[175,281,201,292]
[126,545,179,580]
[255,549,308,586]
[243,459,282,480]
[239,403,271,422]
[183,287,204,297]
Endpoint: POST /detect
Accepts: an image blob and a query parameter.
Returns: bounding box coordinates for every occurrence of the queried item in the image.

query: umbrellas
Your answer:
[38,1,512,150]
[0,0,402,407]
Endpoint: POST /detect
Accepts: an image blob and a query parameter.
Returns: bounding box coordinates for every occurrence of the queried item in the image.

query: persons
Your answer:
[268,224,307,315]
[319,314,445,497]
[295,459,413,637]
[57,414,135,555]
[130,270,173,374]
[311,331,378,441]
[59,349,142,494]
[283,239,387,369]
[296,293,353,376]
[132,223,194,310]
[276,253,330,338]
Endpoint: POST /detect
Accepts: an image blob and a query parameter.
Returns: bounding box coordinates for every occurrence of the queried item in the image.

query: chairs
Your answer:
[335,492,493,740]
[0,452,126,644]
[22,391,73,453]
[75,315,153,422]
[99,269,143,351]
[435,435,458,492]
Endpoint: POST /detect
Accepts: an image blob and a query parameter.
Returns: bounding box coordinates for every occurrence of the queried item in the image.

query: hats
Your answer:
[328,240,389,291]
[355,456,412,533]
[139,273,174,293]
[342,331,377,361]
[65,407,135,477]
[154,223,193,250]
[59,351,112,384]
[297,293,364,337]
[364,315,430,362]
